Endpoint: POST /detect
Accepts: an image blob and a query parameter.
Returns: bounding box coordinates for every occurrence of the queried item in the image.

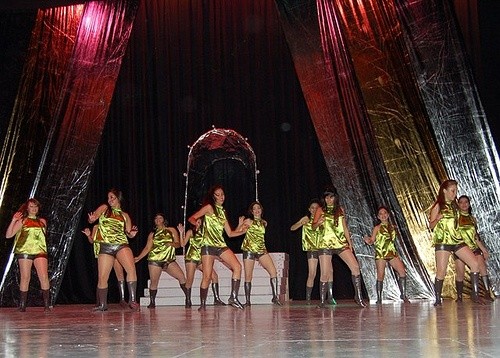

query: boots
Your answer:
[375,280,384,303]
[118,280,127,308]
[471,272,483,302]
[41,289,50,312]
[352,274,368,307]
[480,275,493,299]
[228,278,244,308]
[197,287,208,311]
[185,287,192,308]
[327,282,338,305]
[455,280,464,302]
[147,288,157,308]
[92,287,108,312]
[320,282,328,306]
[305,286,314,304]
[270,277,283,306]
[15,291,29,312]
[397,277,410,303]
[433,277,444,305]
[127,281,141,312]
[244,282,251,306]
[212,283,226,306]
[180,283,192,306]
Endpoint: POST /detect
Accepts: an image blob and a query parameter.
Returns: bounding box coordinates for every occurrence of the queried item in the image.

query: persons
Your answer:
[134,213,192,307]
[82,224,128,309]
[177,219,226,307]
[454,195,494,302]
[430,179,486,306]
[88,189,138,311]
[6,199,50,312]
[312,187,367,307]
[364,207,410,303]
[291,199,337,305]
[235,201,282,306]
[189,184,248,310]
